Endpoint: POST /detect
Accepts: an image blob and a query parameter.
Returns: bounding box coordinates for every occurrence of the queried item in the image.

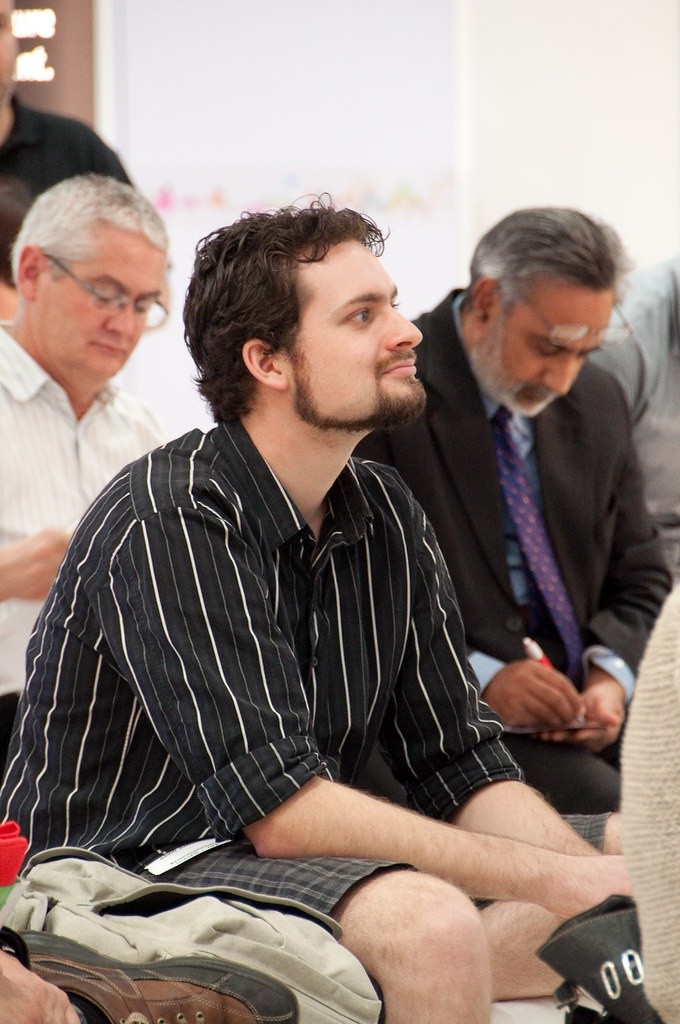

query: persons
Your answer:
[0,925,299,1024]
[0,178,171,729]
[620,586,680,1024]
[0,0,130,314]
[586,253,680,588]
[0,204,633,1023]
[351,208,669,813]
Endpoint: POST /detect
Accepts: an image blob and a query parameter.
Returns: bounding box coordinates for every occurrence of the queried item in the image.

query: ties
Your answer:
[490,401,584,692]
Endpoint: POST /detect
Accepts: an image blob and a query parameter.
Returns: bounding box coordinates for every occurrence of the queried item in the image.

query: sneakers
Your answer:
[14,931,299,1024]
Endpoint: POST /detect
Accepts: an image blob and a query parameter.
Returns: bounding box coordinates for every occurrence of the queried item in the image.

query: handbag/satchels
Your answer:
[1,846,383,1024]
[534,894,666,1024]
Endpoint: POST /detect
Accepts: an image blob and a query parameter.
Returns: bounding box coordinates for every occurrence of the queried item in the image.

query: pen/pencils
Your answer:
[519,636,584,723]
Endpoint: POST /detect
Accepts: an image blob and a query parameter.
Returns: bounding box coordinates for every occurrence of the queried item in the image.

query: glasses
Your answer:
[42,253,168,329]
[516,284,633,347]
